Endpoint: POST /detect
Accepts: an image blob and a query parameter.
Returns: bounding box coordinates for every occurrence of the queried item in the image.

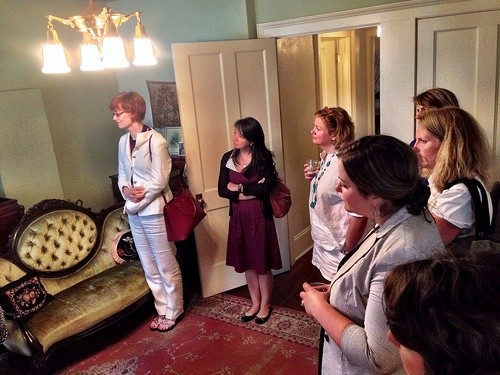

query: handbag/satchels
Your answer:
[270,179,292,218]
[162,187,207,243]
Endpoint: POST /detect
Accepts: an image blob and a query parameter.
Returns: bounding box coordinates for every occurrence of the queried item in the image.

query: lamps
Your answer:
[40,0,156,74]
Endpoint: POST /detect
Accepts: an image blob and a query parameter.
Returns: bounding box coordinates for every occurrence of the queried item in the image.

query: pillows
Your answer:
[0,270,55,323]
[112,229,141,265]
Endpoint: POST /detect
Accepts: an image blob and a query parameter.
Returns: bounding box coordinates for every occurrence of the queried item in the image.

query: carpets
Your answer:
[60,293,323,375]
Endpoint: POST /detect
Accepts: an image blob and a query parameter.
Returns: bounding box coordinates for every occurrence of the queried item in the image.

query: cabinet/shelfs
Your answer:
[1,199,26,250]
[109,175,185,200]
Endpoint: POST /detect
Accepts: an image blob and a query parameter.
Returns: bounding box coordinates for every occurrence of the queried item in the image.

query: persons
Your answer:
[410,88,493,257]
[384,256,500,375]
[300,135,447,375]
[303,108,373,282]
[109,91,184,332]
[218,117,282,324]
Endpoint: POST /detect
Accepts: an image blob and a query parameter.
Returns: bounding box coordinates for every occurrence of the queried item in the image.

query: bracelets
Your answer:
[238,184,242,192]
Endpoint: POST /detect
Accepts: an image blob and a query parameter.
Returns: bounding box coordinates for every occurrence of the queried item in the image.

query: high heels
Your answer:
[158,310,184,332]
[149,315,165,331]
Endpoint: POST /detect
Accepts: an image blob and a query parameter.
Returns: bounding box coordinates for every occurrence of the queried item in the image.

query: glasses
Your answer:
[112,111,124,117]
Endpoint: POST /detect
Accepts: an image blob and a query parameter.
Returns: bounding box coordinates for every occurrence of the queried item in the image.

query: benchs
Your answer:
[0,198,153,375]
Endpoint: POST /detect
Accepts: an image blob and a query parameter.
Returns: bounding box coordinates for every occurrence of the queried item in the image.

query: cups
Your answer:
[308,282,328,292]
[305,159,321,178]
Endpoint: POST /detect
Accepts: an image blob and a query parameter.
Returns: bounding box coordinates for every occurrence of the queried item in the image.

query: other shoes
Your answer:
[255,306,273,324]
[241,306,260,321]
